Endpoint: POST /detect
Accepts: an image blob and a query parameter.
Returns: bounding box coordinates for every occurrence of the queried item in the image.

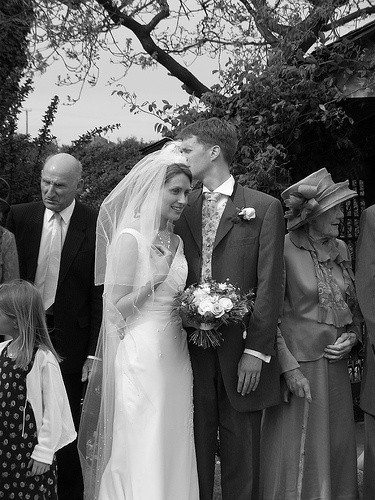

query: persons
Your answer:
[0,277,79,500]
[258,167,364,500]
[1,176,21,284]
[169,116,285,500]
[92,161,200,500]
[356,203,375,499]
[5,154,104,500]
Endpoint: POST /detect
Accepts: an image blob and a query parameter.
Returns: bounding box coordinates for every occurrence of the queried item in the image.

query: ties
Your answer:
[196,187,222,289]
[40,211,63,311]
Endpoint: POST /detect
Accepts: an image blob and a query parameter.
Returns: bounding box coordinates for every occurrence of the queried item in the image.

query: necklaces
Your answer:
[156,231,171,251]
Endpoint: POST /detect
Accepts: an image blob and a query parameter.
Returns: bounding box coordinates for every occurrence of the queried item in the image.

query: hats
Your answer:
[279,168,359,232]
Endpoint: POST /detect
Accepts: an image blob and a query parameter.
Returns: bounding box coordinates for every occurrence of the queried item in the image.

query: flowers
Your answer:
[172,278,254,350]
[232,208,256,222]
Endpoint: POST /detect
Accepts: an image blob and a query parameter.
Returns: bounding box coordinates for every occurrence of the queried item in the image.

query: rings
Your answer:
[251,376,256,378]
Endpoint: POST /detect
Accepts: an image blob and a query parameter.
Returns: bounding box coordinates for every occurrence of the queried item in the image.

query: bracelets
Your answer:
[86,355,103,363]
